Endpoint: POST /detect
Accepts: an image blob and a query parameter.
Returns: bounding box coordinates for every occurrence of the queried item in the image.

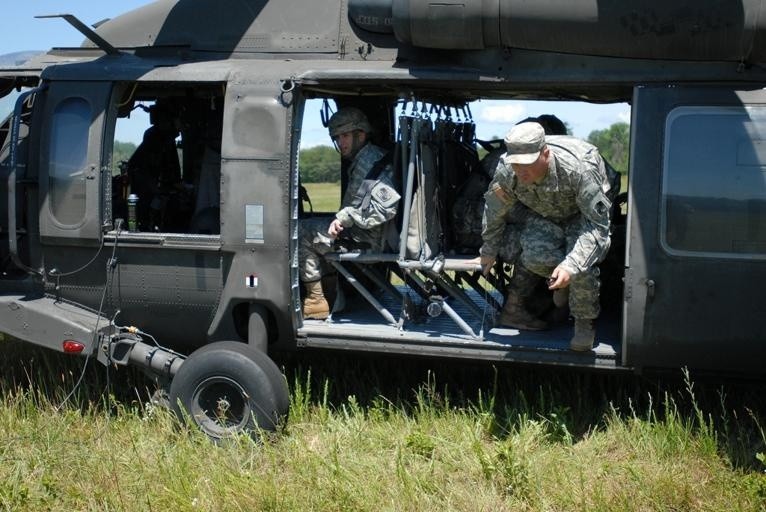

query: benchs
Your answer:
[325,116,496,340]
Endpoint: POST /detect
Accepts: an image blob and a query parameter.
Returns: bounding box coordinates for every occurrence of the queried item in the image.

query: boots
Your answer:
[302,279,329,321]
[570,316,595,352]
[499,294,547,331]
[552,287,570,309]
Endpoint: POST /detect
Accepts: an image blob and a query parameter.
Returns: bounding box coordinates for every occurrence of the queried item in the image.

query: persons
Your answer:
[299,105,401,318]
[450,116,621,351]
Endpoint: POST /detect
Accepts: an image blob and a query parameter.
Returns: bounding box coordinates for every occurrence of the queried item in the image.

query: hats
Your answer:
[503,122,547,164]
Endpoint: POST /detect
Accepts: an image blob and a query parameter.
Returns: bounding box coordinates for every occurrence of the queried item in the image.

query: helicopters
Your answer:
[0,0,766,451]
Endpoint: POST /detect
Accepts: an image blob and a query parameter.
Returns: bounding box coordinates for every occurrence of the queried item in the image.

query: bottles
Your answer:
[126,193,140,233]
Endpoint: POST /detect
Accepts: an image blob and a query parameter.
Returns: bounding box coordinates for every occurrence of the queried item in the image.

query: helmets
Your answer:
[328,107,372,137]
